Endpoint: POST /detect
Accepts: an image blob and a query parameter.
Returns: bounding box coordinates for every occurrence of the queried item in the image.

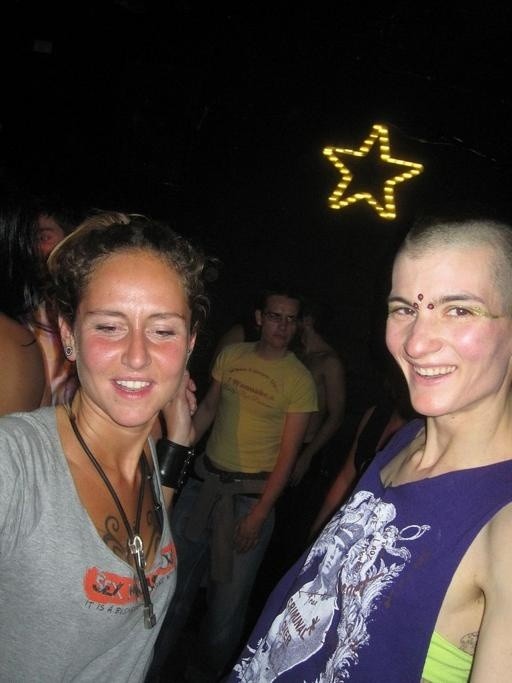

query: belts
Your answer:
[200,453,276,485]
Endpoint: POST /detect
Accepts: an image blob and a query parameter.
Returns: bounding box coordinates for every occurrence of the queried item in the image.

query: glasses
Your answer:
[264,311,297,322]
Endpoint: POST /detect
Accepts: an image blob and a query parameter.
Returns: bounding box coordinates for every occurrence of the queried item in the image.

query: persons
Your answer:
[1,196,511,683]
